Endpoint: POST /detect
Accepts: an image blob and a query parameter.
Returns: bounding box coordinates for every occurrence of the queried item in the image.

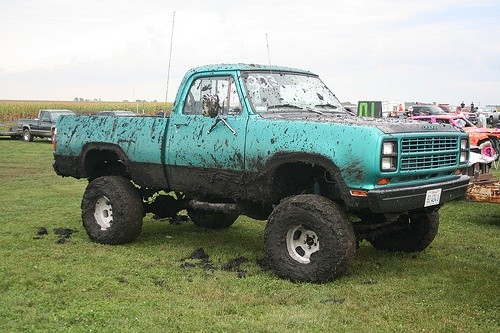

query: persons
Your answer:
[156,109,165,118]
[392,101,500,131]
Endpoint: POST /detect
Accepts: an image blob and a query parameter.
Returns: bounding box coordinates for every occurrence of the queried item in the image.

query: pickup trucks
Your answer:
[16,109,76,142]
[396,102,499,182]
[52,62,470,284]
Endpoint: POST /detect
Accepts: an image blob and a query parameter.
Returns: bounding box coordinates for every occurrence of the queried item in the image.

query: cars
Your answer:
[99,110,136,117]
